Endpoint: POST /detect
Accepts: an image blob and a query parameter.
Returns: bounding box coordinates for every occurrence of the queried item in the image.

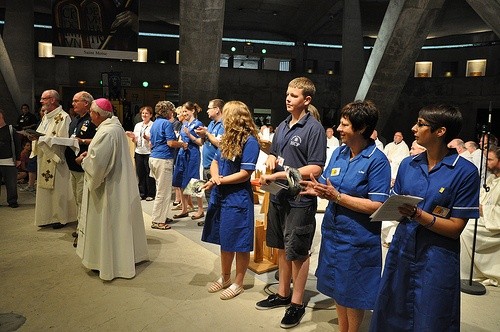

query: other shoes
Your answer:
[191,212,204,219]
[220,283,244,300]
[208,279,232,293]
[53,222,69,229]
[173,212,188,218]
[146,196,153,201]
[171,204,182,210]
[150,222,171,229]
[187,208,194,213]
[140,197,146,200]
[72,232,78,237]
[10,203,18,208]
[166,218,173,222]
[198,220,205,226]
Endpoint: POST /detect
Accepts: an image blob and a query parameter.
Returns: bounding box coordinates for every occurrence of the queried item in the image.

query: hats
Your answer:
[92,98,114,117]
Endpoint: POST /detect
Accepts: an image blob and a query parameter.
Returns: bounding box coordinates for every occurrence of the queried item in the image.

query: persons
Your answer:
[255,126,500,287]
[200,101,261,299]
[131,99,225,226]
[149,101,188,229]
[298,100,390,332]
[259,105,318,281]
[64,91,100,244]
[0,105,45,208]
[76,99,149,279]
[30,90,71,229]
[369,104,480,332]
[256,77,323,328]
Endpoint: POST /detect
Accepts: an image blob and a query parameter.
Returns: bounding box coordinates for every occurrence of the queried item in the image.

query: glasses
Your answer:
[416,121,432,128]
[40,97,52,101]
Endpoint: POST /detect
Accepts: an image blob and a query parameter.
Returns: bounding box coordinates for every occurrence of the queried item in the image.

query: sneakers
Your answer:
[255,292,292,310]
[280,303,306,329]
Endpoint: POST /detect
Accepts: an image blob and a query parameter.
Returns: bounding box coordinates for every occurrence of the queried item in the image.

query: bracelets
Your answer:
[83,140,85,144]
